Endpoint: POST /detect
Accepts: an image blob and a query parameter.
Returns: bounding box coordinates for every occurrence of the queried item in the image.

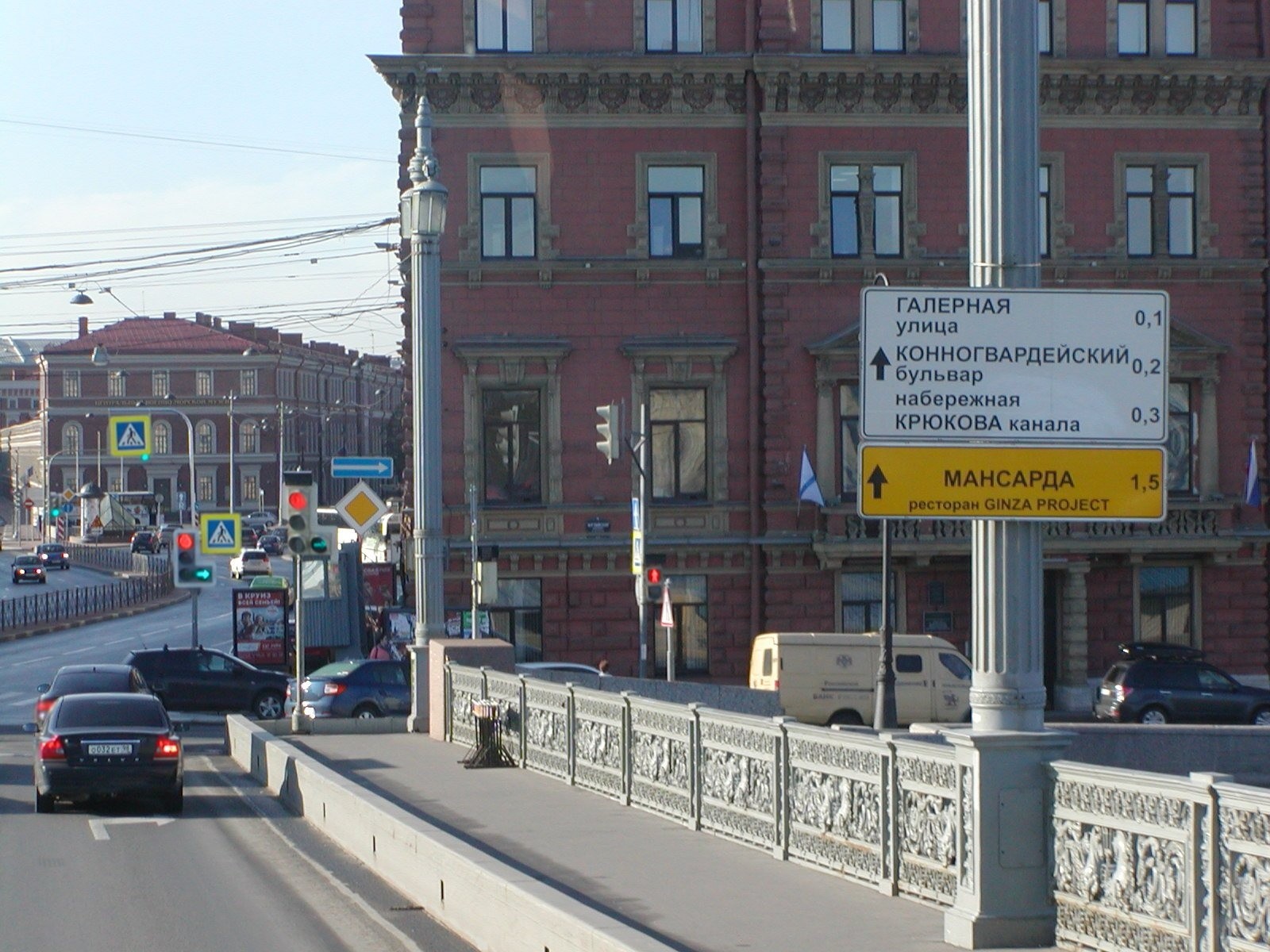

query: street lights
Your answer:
[222,388,239,514]
[276,401,290,525]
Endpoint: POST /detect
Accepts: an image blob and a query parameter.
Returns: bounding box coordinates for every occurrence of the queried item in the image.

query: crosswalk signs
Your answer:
[108,415,152,457]
[201,512,242,556]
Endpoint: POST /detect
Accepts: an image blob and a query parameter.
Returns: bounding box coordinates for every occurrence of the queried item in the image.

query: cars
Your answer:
[246,574,298,613]
[130,531,160,554]
[159,524,184,548]
[33,543,70,570]
[284,658,412,718]
[19,691,186,815]
[10,555,46,584]
[241,511,290,557]
[34,663,167,731]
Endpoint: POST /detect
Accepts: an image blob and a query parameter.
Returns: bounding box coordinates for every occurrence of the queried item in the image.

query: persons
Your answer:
[238,611,253,639]
[198,652,213,670]
[251,614,268,639]
[370,635,390,659]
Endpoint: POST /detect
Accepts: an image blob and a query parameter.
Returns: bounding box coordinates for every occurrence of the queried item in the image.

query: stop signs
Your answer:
[23,499,34,509]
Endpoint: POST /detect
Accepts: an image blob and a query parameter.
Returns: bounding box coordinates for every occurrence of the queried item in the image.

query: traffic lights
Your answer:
[172,528,216,590]
[595,405,620,466]
[282,479,338,557]
[645,565,665,587]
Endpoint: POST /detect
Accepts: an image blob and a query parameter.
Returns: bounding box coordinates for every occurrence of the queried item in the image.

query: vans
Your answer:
[747,630,973,727]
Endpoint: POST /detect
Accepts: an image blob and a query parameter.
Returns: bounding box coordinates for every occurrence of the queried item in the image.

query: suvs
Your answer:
[120,647,294,720]
[1091,642,1270,726]
[229,548,273,580]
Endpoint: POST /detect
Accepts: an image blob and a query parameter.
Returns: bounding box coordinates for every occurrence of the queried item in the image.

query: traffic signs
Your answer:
[858,282,1172,444]
[856,440,1171,524]
